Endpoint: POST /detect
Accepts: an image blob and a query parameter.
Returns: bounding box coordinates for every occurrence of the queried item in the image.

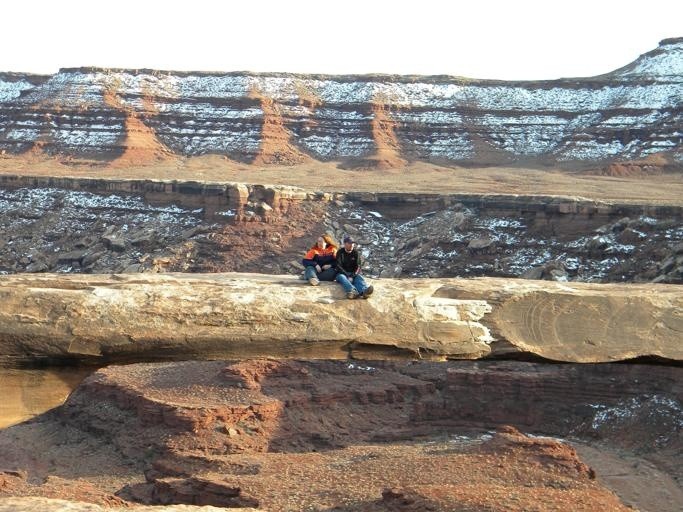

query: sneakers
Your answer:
[348,291,360,299]
[362,286,373,299]
[309,278,320,286]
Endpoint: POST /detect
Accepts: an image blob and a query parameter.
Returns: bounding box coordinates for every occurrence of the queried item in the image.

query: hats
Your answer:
[344,237,357,244]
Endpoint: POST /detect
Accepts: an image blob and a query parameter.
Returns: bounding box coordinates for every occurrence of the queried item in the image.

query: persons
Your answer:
[335,236,375,300]
[302,235,340,286]
